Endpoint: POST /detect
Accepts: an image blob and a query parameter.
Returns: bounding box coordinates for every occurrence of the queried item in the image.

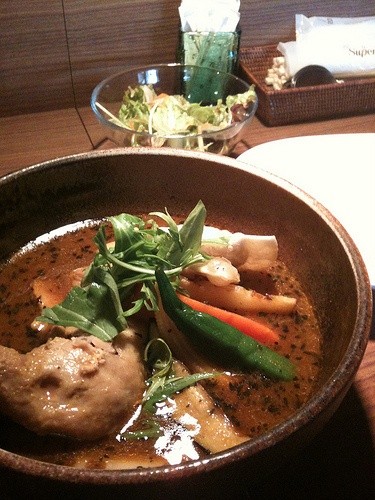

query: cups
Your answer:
[173,22,242,106]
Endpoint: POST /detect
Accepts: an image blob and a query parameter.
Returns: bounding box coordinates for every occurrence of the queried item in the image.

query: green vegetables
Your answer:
[96,82,256,135]
[38,200,222,437]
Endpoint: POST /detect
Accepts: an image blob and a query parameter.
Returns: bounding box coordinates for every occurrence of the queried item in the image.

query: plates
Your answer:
[235,133,375,290]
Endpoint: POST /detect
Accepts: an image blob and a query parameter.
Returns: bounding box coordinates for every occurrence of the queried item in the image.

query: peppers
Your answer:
[154,266,300,382]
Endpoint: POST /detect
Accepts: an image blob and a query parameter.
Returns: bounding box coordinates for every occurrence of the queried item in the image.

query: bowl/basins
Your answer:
[90,63,259,158]
[0,146,372,485]
